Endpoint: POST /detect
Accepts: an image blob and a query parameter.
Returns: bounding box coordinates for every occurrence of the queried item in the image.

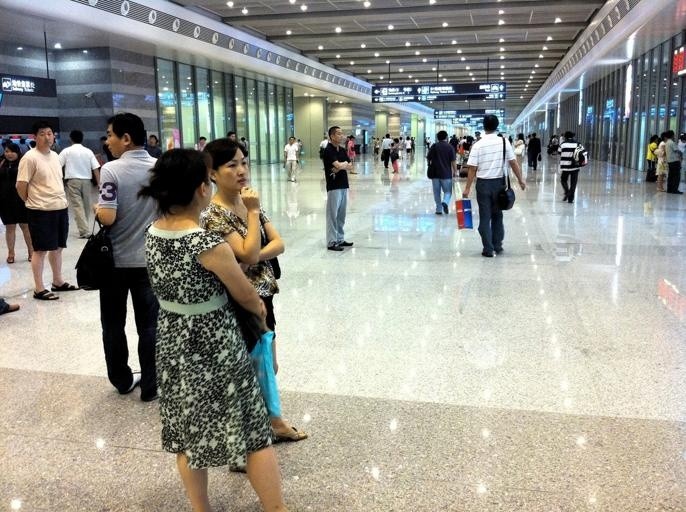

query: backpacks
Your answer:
[573,143,589,166]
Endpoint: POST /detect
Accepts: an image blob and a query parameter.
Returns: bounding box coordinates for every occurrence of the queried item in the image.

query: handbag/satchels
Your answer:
[502,187,514,209]
[248,313,282,417]
[538,154,541,161]
[514,145,524,155]
[266,240,281,280]
[454,170,462,200]
[75,212,114,290]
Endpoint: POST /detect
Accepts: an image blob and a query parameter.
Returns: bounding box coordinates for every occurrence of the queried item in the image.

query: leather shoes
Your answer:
[341,241,353,246]
[328,245,344,251]
[8,304,19,312]
[7,257,13,263]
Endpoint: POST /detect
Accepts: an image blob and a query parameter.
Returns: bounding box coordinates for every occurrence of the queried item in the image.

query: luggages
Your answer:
[646,151,658,182]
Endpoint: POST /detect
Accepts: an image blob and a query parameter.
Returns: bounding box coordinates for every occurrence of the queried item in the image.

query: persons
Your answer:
[557,131,580,203]
[462,114,525,258]
[283,136,303,182]
[323,127,355,252]
[320,132,416,174]
[426,131,459,215]
[644,129,686,194]
[77,112,166,402]
[1,112,247,313]
[134,148,289,512]
[201,135,307,442]
[426,131,565,170]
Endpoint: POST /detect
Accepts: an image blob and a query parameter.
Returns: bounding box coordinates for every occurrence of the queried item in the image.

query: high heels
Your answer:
[272,426,307,442]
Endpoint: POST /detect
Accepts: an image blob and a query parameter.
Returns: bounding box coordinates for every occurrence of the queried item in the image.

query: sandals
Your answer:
[51,282,79,291]
[33,289,59,300]
